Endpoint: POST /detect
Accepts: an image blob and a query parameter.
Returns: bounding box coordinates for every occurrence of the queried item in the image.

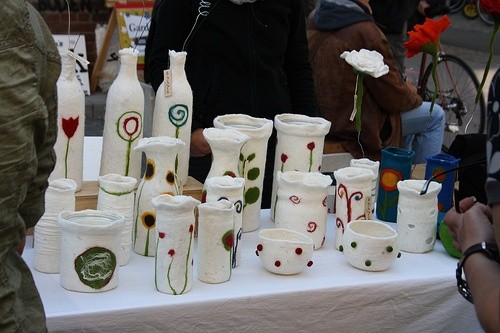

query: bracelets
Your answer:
[456,240,500,304]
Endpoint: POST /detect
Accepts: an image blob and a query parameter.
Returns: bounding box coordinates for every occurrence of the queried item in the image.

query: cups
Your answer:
[204,175,246,267]
[376,147,415,223]
[350,158,380,219]
[271,171,333,250]
[270,113,332,222]
[196,199,235,284]
[396,178,442,253]
[425,152,461,239]
[334,167,375,252]
[131,136,186,257]
[150,194,201,295]
[200,127,252,192]
[57,209,125,293]
[97,174,137,266]
[213,113,273,233]
[32,178,77,274]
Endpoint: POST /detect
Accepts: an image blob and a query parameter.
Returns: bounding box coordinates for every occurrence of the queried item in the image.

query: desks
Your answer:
[21,208,485,333]
[26,176,205,236]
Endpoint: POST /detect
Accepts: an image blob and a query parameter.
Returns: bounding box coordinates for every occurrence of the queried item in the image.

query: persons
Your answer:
[0,0,61,333]
[368,0,450,76]
[444,66,500,333]
[144,0,314,209]
[307,0,446,155]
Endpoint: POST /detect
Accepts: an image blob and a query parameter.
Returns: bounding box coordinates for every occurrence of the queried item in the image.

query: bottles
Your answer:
[97,48,145,195]
[43,53,86,193]
[151,50,193,185]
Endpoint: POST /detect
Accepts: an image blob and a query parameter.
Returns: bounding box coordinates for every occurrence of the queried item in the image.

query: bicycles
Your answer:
[420,55,487,159]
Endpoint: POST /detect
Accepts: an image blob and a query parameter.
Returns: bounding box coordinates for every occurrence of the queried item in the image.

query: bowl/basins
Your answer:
[257,228,314,276]
[342,220,400,272]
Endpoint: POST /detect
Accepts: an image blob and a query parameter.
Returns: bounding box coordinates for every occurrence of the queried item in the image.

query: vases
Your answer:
[48,50,85,192]
[97,175,136,266]
[32,178,77,274]
[151,50,193,185]
[152,193,200,296]
[99,48,144,179]
[196,112,487,284]
[134,136,185,257]
[58,211,126,292]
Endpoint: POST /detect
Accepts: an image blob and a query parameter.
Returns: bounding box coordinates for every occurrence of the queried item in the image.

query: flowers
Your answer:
[464,0,500,134]
[339,48,390,160]
[403,14,452,173]
